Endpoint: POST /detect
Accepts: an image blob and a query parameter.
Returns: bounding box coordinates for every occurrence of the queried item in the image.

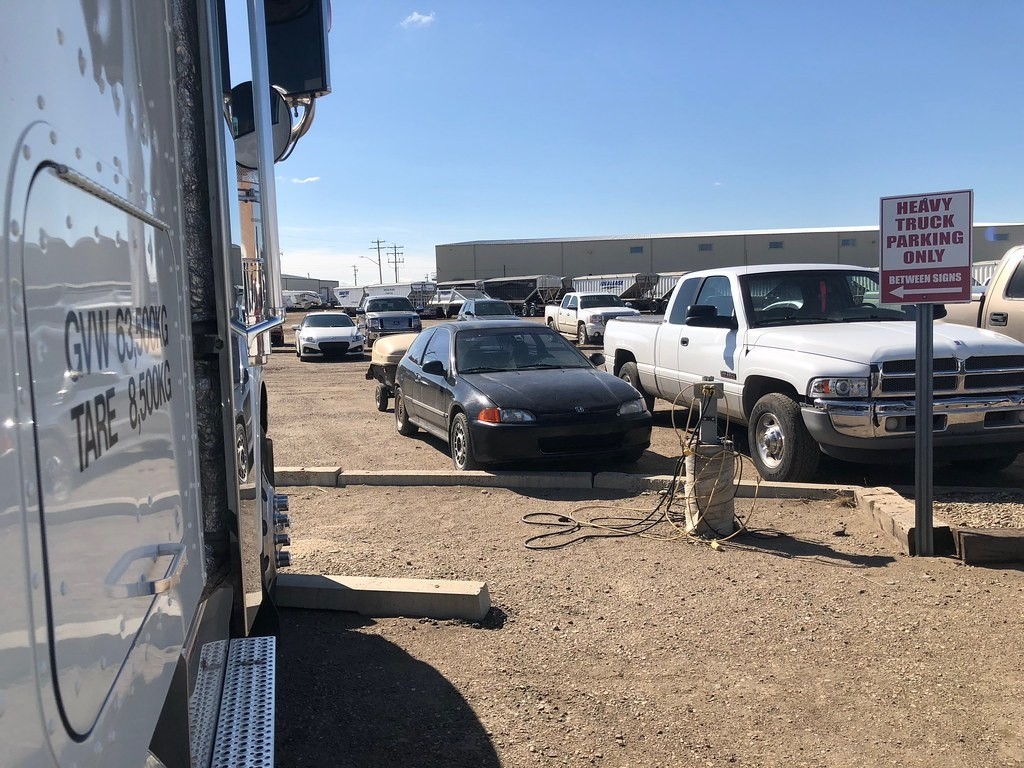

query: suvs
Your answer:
[355,295,422,348]
[457,297,520,320]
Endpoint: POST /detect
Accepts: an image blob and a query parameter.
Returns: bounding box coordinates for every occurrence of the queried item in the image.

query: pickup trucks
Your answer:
[862,245,1024,343]
[545,292,641,345]
[603,263,1024,481]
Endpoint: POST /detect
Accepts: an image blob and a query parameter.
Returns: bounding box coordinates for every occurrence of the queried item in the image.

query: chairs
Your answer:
[392,302,403,310]
[509,341,529,366]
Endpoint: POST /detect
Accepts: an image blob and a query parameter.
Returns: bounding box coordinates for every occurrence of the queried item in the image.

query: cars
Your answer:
[394,319,652,471]
[291,312,365,361]
